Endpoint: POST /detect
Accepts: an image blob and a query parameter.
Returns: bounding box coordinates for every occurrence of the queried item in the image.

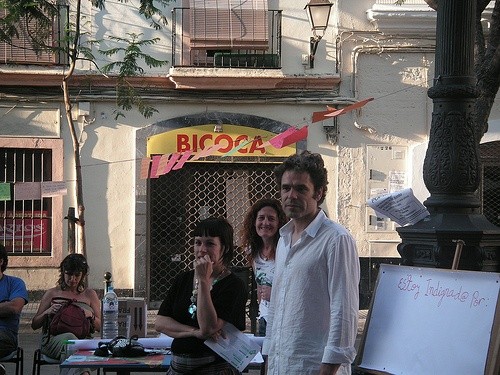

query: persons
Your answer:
[260,150,360,375]
[31,253,101,375]
[239,198,290,337]
[154,218,247,375]
[0,244,28,375]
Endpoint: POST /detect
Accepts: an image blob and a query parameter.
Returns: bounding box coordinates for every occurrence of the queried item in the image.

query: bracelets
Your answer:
[90,314,95,321]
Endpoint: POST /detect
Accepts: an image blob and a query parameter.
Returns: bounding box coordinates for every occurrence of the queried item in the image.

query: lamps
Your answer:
[200,94,223,133]
[304,0,334,69]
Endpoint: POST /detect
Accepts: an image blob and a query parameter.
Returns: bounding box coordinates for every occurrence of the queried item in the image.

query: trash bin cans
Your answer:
[100,294,148,338]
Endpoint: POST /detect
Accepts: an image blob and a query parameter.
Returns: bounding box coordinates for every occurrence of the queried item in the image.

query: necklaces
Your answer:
[189,269,225,319]
[261,250,267,261]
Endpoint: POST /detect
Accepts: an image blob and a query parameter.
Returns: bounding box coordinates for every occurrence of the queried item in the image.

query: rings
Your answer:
[53,309,56,311]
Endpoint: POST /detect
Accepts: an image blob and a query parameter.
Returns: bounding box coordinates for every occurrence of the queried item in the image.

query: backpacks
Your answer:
[49,298,90,339]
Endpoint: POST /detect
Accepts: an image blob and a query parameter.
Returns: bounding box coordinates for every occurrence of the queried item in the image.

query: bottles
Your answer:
[102,286,118,338]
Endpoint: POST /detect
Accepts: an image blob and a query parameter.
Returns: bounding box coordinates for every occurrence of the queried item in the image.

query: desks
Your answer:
[60,334,265,375]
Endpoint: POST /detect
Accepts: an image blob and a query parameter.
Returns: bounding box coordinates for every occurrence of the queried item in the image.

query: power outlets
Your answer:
[301,54,308,65]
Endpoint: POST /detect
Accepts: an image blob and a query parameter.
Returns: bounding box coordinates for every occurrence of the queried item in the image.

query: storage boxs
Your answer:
[118,297,147,338]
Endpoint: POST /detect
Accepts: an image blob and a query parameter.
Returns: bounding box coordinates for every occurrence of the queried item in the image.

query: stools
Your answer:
[32,348,106,375]
[0,347,23,375]
